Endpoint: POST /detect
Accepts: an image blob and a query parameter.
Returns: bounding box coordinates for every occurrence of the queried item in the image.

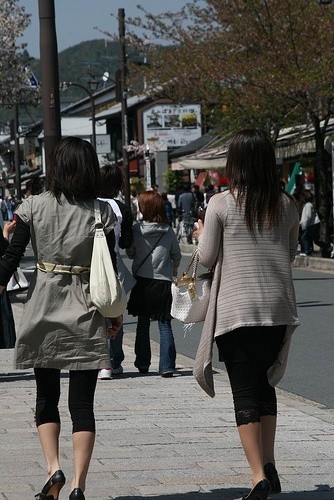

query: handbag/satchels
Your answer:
[190,193,204,217]
[170,248,212,324]
[90,200,128,318]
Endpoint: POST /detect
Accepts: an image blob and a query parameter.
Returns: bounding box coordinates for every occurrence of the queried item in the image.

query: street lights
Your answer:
[58,74,101,151]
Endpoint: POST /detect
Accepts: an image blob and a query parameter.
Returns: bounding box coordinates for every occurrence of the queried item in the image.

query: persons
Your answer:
[98,163,134,379]
[154,181,231,244]
[0,179,43,232]
[125,191,182,376]
[0,218,17,349]
[192,129,301,500]
[287,177,325,257]
[0,137,127,500]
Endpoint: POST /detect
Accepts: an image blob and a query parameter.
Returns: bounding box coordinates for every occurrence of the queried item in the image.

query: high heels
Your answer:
[68,488,85,500]
[34,470,66,500]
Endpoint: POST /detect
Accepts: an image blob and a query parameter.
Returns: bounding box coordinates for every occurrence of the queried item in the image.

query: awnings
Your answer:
[171,118,334,171]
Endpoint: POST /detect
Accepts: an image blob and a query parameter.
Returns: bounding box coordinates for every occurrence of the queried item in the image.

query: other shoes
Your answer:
[242,479,271,500]
[134,362,147,375]
[112,365,123,375]
[265,462,282,494]
[162,372,173,378]
[96,367,113,379]
